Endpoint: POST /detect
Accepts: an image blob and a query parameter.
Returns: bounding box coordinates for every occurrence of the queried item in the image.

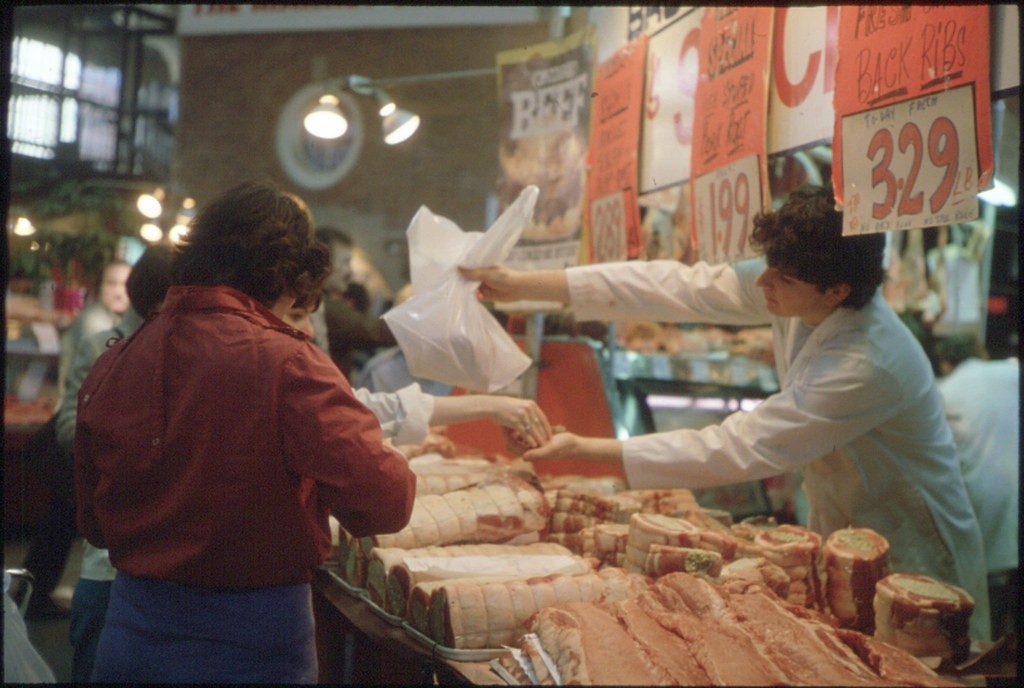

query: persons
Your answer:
[0,180,557,687]
[456,182,1019,655]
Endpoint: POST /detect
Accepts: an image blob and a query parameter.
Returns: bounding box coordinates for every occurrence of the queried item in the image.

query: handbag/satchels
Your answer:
[381,182,540,395]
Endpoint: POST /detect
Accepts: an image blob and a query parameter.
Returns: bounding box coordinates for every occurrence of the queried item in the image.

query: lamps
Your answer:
[304,65,498,146]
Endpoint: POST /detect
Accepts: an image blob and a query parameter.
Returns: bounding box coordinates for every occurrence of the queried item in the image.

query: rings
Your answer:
[525,424,532,432]
[532,416,539,424]
